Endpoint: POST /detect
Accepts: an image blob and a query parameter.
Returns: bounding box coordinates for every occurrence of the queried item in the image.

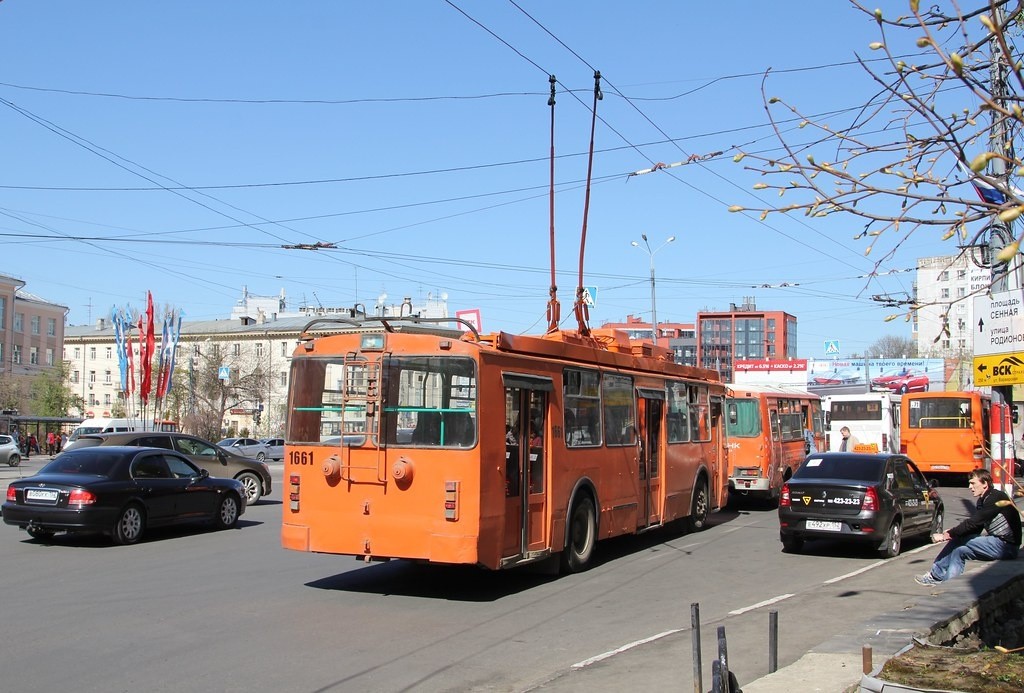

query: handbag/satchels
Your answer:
[46,437,51,444]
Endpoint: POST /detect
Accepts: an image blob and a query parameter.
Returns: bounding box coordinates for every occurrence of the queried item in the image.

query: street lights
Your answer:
[632,234,676,345]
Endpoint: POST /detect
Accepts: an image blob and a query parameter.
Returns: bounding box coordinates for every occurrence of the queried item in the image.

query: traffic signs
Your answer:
[973,288,1024,356]
[972,351,1024,387]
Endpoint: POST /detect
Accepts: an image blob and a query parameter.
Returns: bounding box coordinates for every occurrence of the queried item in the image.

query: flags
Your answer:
[112,290,183,406]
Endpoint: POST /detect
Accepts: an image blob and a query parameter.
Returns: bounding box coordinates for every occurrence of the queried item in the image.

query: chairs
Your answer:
[411,408,475,447]
[517,406,575,432]
[832,411,878,420]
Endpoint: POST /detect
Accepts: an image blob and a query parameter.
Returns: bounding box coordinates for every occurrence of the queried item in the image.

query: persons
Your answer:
[505,406,599,446]
[838,426,859,452]
[914,469,1023,586]
[803,424,818,458]
[847,405,857,417]
[11,425,68,458]
[834,405,847,418]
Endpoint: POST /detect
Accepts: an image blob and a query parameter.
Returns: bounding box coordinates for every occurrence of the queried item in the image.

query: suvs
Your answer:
[50,431,272,506]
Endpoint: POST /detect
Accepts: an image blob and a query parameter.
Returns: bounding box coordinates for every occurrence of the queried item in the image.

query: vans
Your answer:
[62,417,154,449]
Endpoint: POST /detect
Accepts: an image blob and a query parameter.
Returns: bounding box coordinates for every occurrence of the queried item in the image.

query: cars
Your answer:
[1,445,247,546]
[778,443,945,559]
[0,435,21,467]
[870,367,930,396]
[201,438,268,462]
[259,438,285,461]
[396,428,416,444]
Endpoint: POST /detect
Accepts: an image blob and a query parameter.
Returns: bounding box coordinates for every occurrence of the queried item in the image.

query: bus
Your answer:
[281,69,738,577]
[900,392,1018,489]
[698,383,830,510]
[319,417,379,445]
[823,393,920,455]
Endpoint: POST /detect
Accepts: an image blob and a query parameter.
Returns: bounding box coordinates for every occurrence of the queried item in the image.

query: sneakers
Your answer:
[915,571,942,587]
